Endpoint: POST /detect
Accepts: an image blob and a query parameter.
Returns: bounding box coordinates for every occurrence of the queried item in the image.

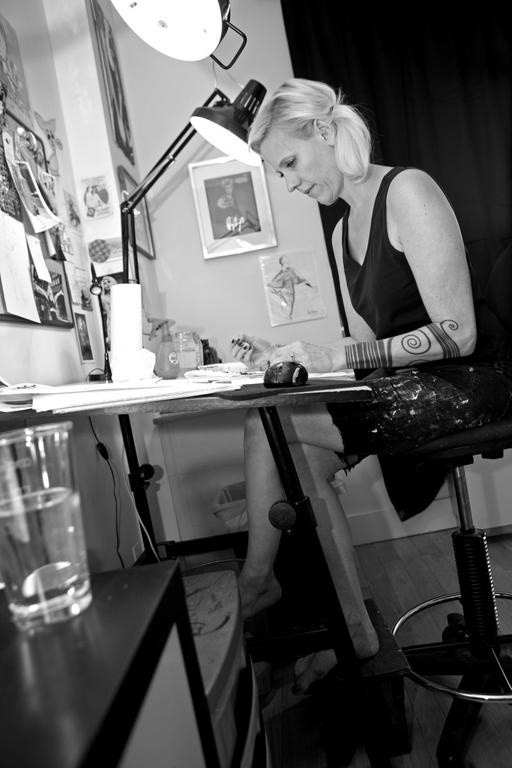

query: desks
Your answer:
[0,372,412,761]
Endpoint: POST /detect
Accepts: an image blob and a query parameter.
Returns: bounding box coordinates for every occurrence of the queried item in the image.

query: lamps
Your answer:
[97,77,270,383]
[110,0,247,74]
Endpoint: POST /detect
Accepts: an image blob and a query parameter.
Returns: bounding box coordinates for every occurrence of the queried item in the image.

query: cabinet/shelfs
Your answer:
[0,551,219,766]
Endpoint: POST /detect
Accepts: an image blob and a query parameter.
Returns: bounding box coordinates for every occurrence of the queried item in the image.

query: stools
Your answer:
[394,415,512,768]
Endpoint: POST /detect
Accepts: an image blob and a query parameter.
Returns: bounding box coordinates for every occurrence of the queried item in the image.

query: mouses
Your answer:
[264,361,309,388]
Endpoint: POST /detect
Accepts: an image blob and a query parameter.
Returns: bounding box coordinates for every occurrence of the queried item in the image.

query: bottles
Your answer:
[173,332,204,375]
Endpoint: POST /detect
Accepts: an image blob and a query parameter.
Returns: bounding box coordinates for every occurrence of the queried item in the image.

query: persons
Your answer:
[231,173,260,231]
[84,186,107,208]
[95,184,109,203]
[230,77,478,694]
[214,176,234,218]
[100,276,117,346]
[266,253,315,320]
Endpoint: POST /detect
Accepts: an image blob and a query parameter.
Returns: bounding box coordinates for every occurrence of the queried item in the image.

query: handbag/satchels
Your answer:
[369,361,508,444]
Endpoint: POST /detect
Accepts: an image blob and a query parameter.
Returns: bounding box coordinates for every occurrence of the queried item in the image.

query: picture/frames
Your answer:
[92,1,135,166]
[187,155,278,260]
[118,166,156,260]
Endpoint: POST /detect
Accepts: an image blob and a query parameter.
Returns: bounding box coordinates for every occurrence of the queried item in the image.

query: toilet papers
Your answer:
[109,283,157,384]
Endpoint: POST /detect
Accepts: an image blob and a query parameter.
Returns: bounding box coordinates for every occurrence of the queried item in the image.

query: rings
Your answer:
[289,352,296,363]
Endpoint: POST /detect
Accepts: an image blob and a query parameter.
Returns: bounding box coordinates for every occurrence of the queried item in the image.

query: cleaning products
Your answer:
[147,315,181,380]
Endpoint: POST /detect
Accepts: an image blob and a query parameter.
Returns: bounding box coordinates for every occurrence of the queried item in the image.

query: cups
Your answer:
[0,420,94,635]
